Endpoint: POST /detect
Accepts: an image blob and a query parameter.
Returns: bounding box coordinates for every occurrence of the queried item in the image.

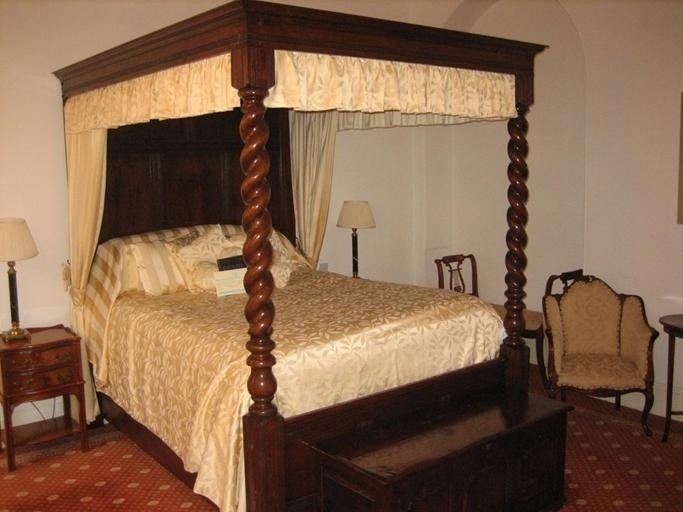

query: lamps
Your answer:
[539,270,660,440]
[431,252,479,305]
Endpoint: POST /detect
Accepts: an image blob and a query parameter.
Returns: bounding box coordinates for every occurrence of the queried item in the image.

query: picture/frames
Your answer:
[654,310,682,445]
[480,298,549,396]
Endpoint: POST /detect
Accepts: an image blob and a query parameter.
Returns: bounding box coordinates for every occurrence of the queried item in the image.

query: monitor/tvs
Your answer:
[212,255,247,296]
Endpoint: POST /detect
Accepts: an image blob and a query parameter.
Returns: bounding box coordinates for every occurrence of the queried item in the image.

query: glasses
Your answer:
[0,214,39,346]
[333,199,375,280]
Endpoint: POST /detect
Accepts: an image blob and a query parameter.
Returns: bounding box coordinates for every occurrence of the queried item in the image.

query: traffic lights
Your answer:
[81,222,318,300]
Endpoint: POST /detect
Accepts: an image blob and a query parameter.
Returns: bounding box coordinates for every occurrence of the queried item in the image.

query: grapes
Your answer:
[0,323,91,473]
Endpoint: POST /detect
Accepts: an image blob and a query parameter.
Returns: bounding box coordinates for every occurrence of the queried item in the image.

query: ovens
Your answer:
[42,1,579,509]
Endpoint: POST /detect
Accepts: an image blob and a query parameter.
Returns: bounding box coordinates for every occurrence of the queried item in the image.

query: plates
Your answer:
[298,381,576,512]
